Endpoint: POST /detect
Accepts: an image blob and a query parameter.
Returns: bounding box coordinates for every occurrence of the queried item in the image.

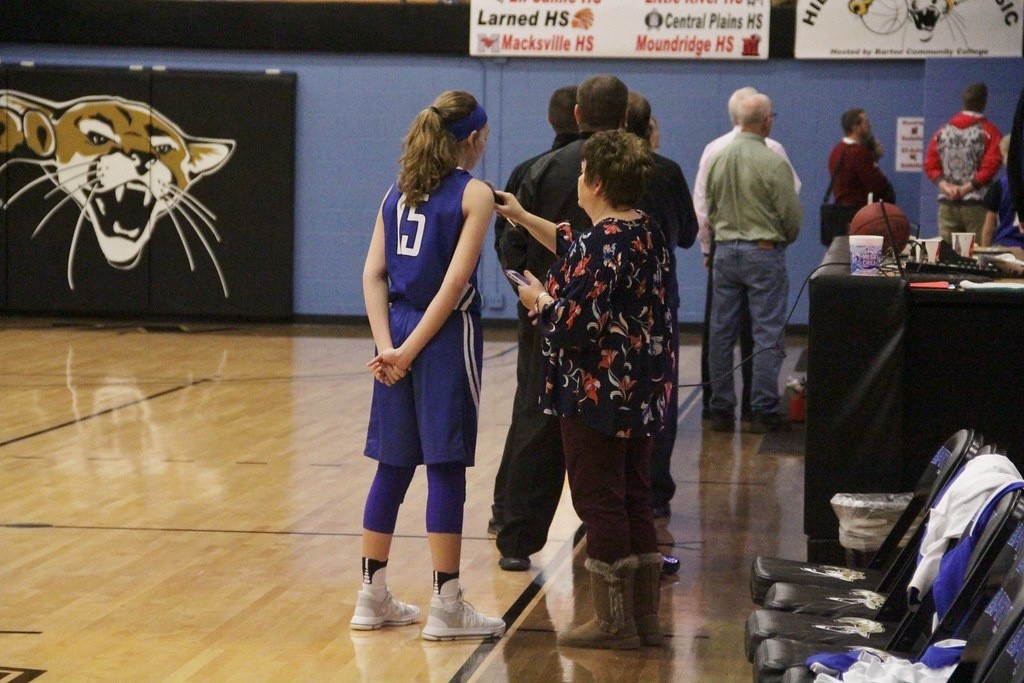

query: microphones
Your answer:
[484,181,526,239]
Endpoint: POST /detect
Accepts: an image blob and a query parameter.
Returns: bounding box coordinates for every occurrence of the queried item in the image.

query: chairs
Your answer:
[745,428,1024,683]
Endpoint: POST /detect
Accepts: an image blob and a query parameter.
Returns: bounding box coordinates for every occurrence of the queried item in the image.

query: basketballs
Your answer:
[849,202,910,255]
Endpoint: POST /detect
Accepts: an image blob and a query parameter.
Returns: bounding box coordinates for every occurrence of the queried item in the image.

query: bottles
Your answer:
[787,376,806,397]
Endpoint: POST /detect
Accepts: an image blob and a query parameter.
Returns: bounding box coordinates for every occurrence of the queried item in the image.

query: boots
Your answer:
[634,554,664,645]
[558,557,640,649]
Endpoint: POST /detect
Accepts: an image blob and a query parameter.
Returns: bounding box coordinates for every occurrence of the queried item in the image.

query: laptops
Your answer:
[877,198,993,283]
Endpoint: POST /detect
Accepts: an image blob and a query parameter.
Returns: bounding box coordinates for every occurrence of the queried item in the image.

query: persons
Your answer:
[864,135,885,160]
[349,89,507,639]
[979,134,1023,251]
[1006,91,1024,232]
[693,86,802,432]
[487,74,700,574]
[493,127,670,647]
[924,84,1004,244]
[829,106,890,209]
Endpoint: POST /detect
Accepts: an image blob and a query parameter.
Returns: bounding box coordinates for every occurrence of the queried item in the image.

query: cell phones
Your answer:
[505,269,530,287]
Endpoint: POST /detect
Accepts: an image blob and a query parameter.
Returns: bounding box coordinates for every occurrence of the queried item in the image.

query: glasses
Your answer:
[764,113,776,122]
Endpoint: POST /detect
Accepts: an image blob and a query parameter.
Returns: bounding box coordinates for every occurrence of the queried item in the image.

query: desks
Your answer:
[804,236,1024,569]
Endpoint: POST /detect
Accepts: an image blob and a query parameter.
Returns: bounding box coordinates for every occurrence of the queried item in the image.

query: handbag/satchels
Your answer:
[820,203,861,247]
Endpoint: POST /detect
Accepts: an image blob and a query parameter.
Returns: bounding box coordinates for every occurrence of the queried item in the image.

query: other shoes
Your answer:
[488,519,504,535]
[713,416,735,431]
[742,412,752,422]
[500,556,531,571]
[751,412,793,434]
[702,408,710,418]
[661,553,680,575]
[652,504,671,520]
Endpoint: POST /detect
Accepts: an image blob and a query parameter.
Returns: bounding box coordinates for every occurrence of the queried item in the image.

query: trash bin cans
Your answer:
[831,492,913,569]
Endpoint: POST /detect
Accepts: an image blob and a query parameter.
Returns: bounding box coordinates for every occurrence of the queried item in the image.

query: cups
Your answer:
[916,240,940,265]
[952,233,976,258]
[850,235,884,276]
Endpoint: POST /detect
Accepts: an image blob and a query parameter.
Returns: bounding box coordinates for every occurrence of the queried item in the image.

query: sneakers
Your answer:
[350,591,420,630]
[421,596,505,640]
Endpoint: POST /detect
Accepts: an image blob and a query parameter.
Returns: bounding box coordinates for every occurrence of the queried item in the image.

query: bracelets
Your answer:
[534,292,549,313]
